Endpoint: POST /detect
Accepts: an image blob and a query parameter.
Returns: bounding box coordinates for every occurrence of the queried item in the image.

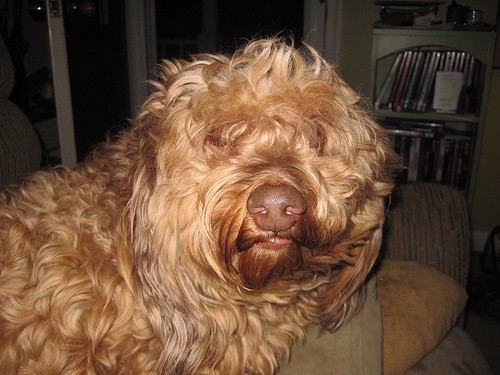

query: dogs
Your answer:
[1,21,410,375]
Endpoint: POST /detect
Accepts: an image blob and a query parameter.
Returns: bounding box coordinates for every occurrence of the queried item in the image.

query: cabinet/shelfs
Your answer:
[368,28,500,255]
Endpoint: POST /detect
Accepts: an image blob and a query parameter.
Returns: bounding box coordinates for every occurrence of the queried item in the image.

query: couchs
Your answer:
[0,37,470,375]
